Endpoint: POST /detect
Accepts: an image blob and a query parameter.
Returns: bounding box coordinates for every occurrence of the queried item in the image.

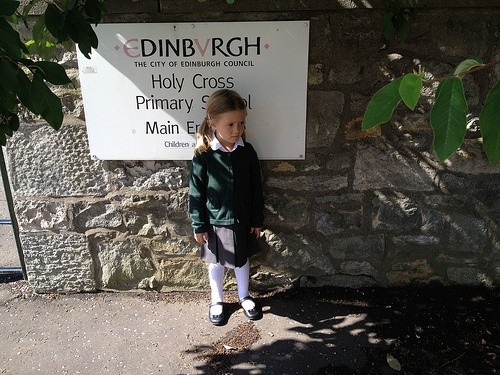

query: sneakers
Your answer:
[238,295,261,319]
[208,301,225,325]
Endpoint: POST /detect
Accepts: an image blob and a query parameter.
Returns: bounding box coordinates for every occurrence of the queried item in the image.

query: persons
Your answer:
[188,88,266,324]
